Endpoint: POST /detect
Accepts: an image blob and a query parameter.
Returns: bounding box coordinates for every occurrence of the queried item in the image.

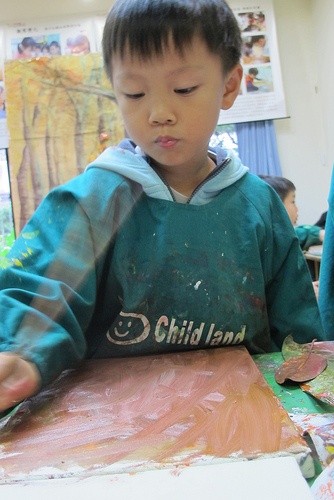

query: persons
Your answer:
[238,11,270,92]
[0,0,326,412]
[255,174,326,250]
[16,36,62,56]
